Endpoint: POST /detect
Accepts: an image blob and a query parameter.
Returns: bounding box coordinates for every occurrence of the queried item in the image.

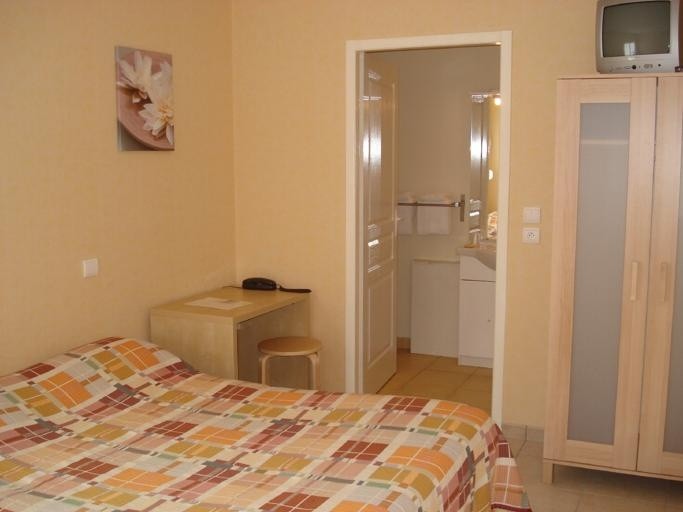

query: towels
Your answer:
[394,189,415,240]
[417,193,453,238]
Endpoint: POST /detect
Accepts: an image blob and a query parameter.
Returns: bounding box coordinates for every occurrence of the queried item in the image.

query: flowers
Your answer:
[116,48,176,146]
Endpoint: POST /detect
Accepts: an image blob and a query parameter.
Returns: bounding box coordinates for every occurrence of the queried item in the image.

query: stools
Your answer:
[256,334,325,393]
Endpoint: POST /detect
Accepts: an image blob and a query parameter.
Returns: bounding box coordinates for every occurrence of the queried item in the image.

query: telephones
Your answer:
[242,277,277,291]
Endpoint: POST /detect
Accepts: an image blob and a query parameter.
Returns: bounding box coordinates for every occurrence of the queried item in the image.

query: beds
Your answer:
[3,337,533,511]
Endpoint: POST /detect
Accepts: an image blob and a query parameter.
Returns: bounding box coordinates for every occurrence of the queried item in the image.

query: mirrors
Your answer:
[469,92,500,250]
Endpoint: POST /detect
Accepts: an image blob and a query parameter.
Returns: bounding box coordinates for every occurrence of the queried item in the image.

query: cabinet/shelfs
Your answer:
[541,73,683,501]
[456,255,497,368]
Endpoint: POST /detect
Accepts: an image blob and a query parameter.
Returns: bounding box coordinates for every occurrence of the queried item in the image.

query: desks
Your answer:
[146,284,311,383]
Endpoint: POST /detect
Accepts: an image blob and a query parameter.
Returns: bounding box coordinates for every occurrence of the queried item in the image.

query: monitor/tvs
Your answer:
[595,0,680,74]
